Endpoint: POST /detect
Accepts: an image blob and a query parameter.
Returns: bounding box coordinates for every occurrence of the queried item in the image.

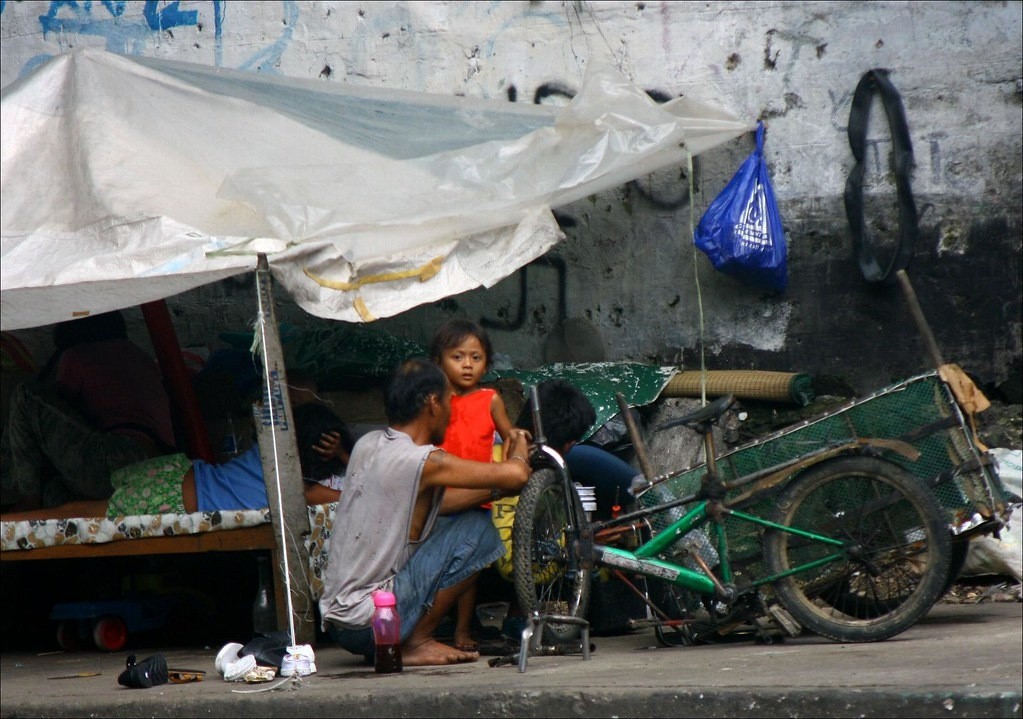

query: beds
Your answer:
[0,503,336,634]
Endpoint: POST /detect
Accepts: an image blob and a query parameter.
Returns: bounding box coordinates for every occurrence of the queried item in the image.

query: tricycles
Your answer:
[512,357,1020,663]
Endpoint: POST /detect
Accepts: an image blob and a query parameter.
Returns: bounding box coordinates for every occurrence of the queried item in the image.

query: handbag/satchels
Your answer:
[694,123,788,282]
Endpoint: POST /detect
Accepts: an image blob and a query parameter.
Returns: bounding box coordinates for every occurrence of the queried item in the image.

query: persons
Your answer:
[0,402,352,522]
[428,318,599,648]
[319,358,530,666]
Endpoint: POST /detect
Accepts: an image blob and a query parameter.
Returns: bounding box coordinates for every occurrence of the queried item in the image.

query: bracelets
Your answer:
[335,447,345,460]
[511,455,525,463]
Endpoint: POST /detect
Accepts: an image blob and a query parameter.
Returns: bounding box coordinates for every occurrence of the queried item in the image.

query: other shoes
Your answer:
[118,653,168,688]
[168,668,207,683]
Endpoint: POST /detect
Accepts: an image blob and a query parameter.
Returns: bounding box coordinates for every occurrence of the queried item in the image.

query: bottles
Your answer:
[253,590,272,632]
[372,592,402,674]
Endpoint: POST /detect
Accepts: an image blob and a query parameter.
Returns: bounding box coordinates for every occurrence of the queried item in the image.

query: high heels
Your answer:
[238,631,292,677]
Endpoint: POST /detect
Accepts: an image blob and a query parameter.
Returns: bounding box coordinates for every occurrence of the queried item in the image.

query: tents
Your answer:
[0,46,761,677]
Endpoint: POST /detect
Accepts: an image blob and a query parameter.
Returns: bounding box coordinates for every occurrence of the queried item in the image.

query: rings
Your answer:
[518,431,525,435]
